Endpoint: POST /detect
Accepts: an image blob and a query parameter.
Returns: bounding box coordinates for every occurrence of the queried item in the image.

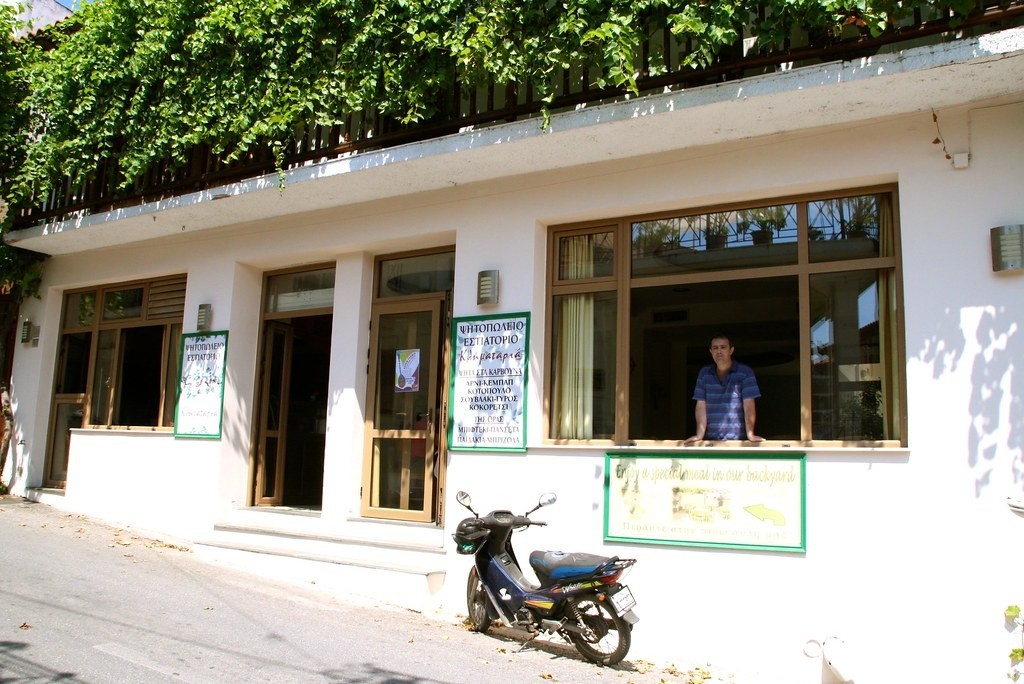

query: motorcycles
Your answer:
[449,491,640,667]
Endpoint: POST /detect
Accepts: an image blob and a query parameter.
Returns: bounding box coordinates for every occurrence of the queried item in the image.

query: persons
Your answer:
[683,333,767,445]
[396,394,440,522]
